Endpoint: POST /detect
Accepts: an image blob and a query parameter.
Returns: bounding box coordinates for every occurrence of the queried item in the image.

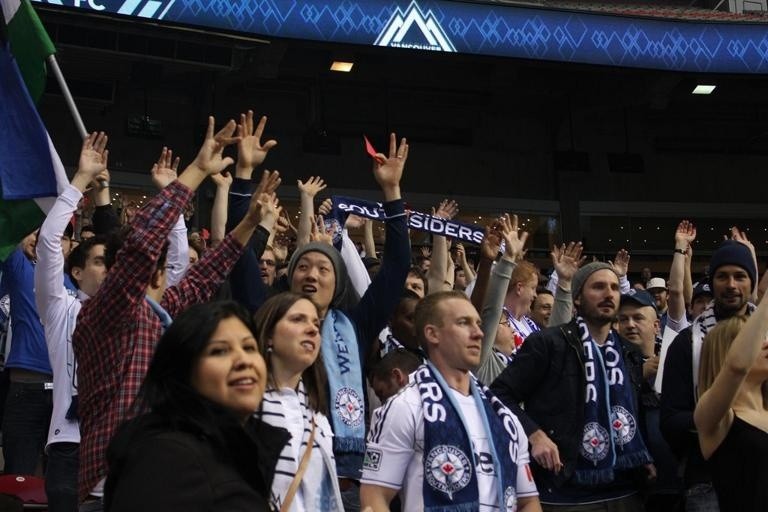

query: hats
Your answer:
[645,277,669,292]
[286,241,348,309]
[573,262,620,300]
[620,291,661,319]
[691,282,715,306]
[709,240,756,294]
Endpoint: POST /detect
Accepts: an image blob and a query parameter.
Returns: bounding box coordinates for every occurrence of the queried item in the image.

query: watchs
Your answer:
[673,249,688,255]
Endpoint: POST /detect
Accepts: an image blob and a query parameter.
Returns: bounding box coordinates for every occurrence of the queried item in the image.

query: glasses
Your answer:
[499,320,515,328]
[259,258,277,266]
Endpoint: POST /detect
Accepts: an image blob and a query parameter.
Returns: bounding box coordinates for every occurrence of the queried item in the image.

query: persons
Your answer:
[252,292,347,512]
[530,288,555,328]
[659,234,738,340]
[103,299,292,511]
[634,266,653,291]
[549,240,570,295]
[258,198,382,263]
[487,261,658,511]
[652,219,760,419]
[505,257,543,358]
[693,288,768,512]
[33,131,191,510]
[70,116,282,512]
[420,233,455,293]
[645,276,670,317]
[660,239,760,512]
[225,110,412,498]
[80,223,102,240]
[357,289,543,511]
[209,170,328,300]
[616,289,667,512]
[1,218,78,476]
[453,243,475,298]
[469,212,588,389]
[366,347,423,408]
[404,196,461,299]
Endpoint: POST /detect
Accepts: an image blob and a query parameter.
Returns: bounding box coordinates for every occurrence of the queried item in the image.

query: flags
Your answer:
[0,0,58,103]
[0,22,73,201]
